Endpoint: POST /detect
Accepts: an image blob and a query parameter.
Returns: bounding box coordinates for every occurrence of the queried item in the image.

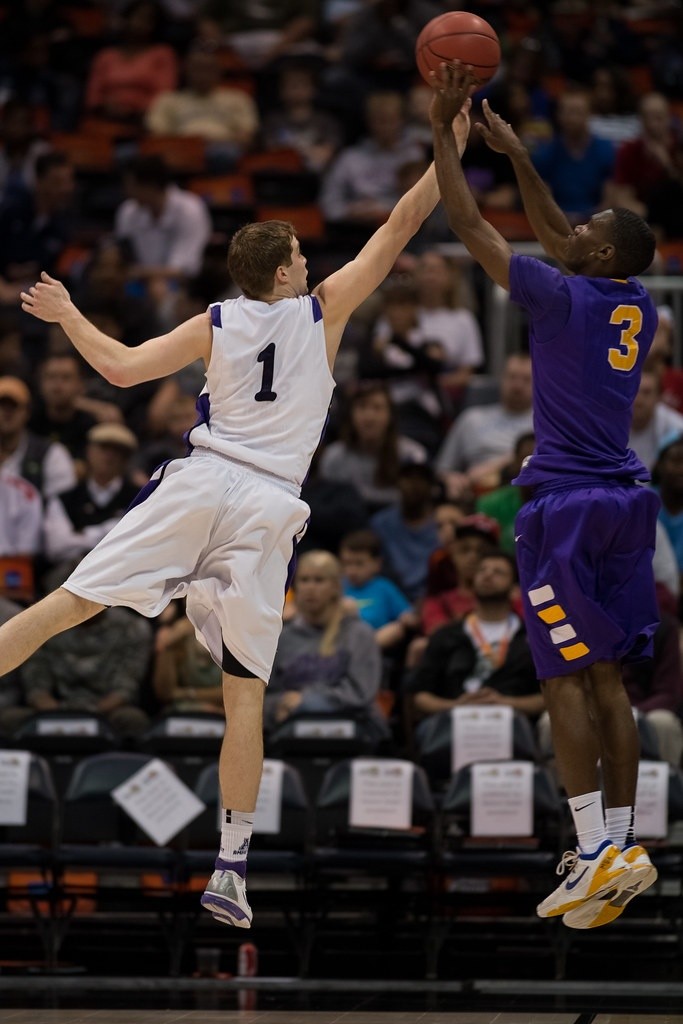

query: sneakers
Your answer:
[537,840,632,918]
[200,857,252,928]
[563,842,658,929]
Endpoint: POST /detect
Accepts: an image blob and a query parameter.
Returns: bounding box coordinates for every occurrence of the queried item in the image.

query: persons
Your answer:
[0,0,683,849]
[429,57,660,931]
[0,88,471,930]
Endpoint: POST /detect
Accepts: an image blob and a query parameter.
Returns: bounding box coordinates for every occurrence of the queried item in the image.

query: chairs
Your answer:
[0,127,683,969]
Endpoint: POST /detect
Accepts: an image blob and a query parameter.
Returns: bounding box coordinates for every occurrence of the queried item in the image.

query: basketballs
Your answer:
[415,10,501,90]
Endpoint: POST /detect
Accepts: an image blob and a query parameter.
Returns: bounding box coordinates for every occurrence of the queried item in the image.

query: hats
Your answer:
[85,421,138,450]
[456,513,500,546]
[0,378,29,406]
